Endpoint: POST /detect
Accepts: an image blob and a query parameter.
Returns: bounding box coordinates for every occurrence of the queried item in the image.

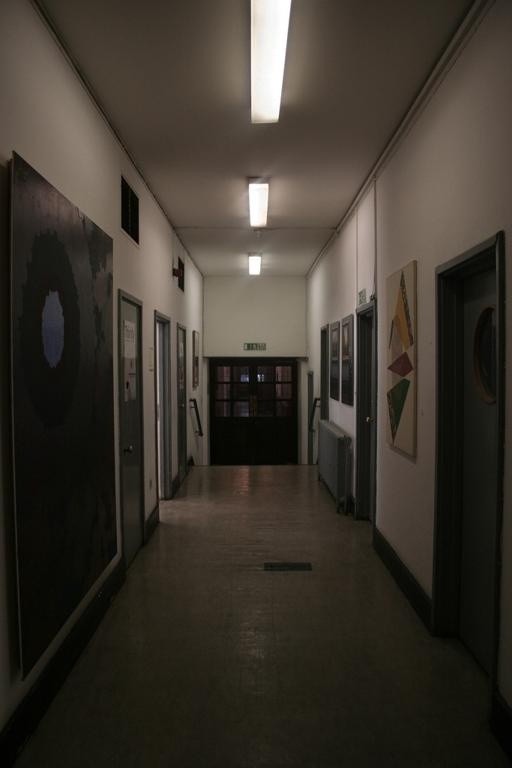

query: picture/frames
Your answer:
[330,313,354,407]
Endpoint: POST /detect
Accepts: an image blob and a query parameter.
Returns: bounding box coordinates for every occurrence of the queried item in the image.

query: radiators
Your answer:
[317,420,352,515]
